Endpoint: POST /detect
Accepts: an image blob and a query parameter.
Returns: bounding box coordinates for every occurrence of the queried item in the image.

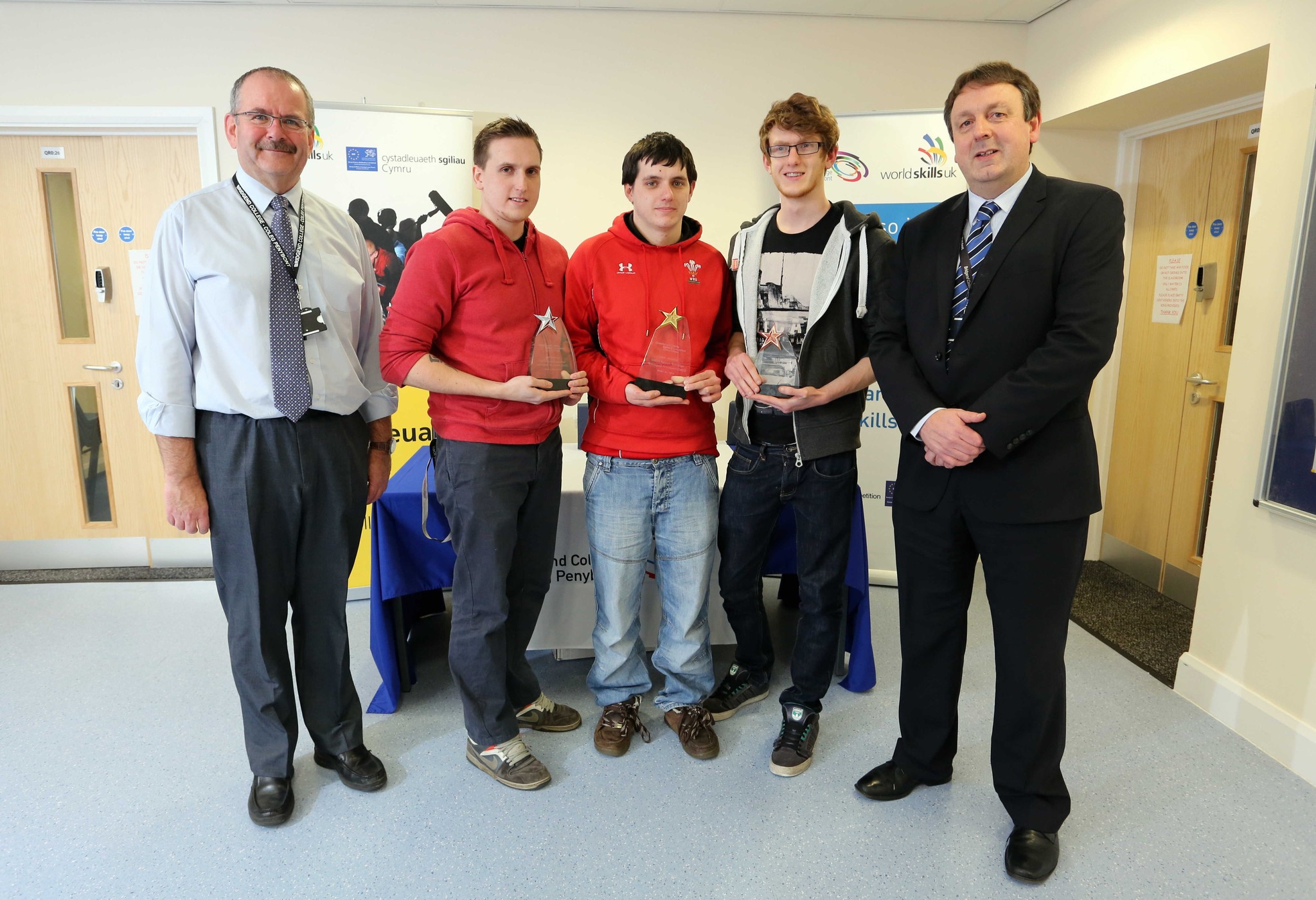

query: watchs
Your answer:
[370,437,397,454]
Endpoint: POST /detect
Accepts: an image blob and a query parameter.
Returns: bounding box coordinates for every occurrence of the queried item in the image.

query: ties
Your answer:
[945,201,1001,374]
[269,196,312,424]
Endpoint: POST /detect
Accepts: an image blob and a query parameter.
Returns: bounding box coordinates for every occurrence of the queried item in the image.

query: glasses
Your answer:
[766,142,825,157]
[233,112,311,133]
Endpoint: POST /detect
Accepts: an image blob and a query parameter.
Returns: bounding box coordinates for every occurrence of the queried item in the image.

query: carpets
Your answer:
[1068,559,1195,689]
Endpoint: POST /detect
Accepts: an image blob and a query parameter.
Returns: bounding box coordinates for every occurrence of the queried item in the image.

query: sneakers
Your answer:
[513,692,582,732]
[665,700,719,759]
[465,732,555,790]
[769,702,820,776]
[594,694,651,756]
[701,659,770,723]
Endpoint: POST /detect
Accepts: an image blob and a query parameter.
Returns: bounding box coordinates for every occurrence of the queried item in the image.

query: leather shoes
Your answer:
[248,765,294,826]
[313,745,387,792]
[855,761,955,802]
[1004,827,1059,881]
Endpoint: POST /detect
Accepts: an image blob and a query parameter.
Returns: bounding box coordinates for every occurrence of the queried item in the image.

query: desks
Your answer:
[366,446,880,717]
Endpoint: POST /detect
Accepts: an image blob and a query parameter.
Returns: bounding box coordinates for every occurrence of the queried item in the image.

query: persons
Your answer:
[561,132,734,762]
[348,199,428,321]
[865,64,1124,886]
[384,119,589,790]
[137,64,399,826]
[722,92,891,777]
